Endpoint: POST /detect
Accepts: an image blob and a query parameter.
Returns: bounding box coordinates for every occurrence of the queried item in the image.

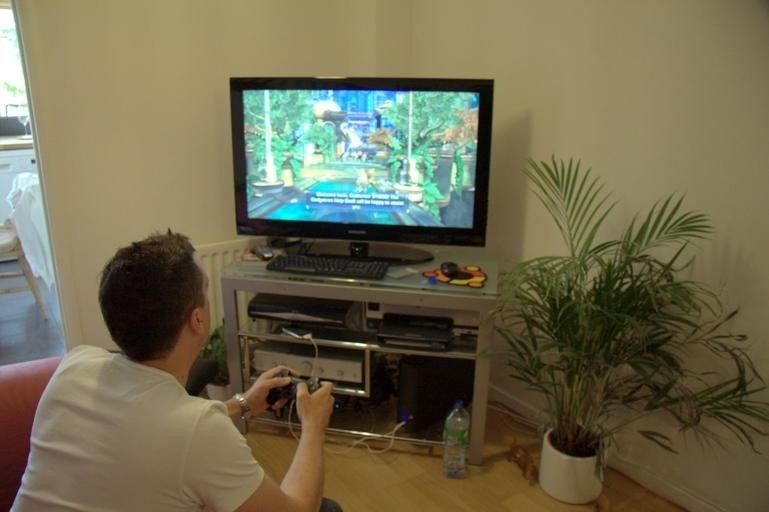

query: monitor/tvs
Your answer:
[230,77,493,266]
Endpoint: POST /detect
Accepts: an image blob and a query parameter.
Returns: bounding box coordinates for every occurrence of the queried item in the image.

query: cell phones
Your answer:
[282,324,313,339]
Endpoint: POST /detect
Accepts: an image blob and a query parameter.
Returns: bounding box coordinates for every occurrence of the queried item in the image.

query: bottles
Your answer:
[443,401,471,479]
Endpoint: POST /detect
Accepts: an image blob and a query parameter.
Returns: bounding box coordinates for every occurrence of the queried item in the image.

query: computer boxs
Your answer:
[396,356,475,433]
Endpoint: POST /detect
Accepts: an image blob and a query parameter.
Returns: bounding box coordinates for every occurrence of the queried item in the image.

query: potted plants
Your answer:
[200,319,261,403]
[471,153,768,505]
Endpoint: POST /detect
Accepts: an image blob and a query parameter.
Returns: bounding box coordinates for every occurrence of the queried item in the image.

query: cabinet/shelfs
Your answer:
[220,247,500,466]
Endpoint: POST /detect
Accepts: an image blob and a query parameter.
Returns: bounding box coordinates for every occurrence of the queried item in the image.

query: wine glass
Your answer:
[16,107,32,140]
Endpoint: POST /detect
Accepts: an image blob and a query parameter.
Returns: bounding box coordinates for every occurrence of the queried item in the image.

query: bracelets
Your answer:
[232,392,251,425]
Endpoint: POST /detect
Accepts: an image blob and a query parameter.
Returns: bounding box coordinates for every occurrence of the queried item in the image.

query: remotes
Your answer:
[250,244,273,260]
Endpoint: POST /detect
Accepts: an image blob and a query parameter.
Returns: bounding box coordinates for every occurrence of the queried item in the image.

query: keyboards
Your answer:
[266,254,391,280]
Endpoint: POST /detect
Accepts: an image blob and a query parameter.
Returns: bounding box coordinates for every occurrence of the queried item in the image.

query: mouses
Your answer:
[441,262,458,278]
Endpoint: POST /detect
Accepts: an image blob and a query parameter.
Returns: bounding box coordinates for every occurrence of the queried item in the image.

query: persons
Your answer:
[7,226,344,512]
